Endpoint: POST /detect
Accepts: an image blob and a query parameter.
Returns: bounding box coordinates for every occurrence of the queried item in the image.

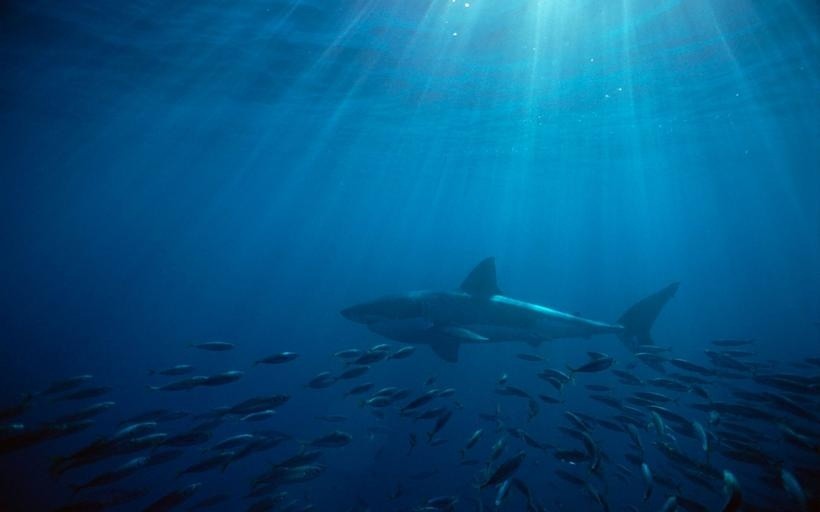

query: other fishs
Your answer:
[0,340,820,511]
